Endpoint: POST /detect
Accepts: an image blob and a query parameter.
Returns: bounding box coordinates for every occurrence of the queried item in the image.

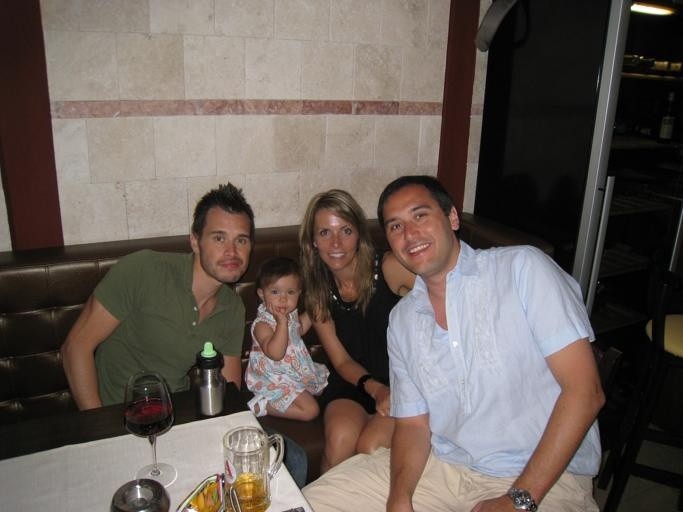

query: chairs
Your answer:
[596,268,683,511]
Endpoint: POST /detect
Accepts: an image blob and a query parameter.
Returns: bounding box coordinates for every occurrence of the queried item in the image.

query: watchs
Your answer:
[506,487,537,512]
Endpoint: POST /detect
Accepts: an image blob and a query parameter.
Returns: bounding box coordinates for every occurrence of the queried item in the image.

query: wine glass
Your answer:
[122,371,177,489]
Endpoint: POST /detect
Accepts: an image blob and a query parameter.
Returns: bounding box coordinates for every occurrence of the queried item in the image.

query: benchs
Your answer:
[0,209,555,479]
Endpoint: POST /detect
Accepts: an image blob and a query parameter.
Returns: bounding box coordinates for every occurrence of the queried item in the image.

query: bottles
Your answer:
[196,342,225,415]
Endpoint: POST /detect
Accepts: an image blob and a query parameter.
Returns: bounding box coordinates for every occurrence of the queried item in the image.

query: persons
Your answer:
[240,257,331,421]
[60,181,308,491]
[297,190,417,476]
[301,176,606,512]
[357,374,374,393]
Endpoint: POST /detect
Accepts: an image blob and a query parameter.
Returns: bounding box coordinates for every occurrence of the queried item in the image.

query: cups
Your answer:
[222,426,285,511]
